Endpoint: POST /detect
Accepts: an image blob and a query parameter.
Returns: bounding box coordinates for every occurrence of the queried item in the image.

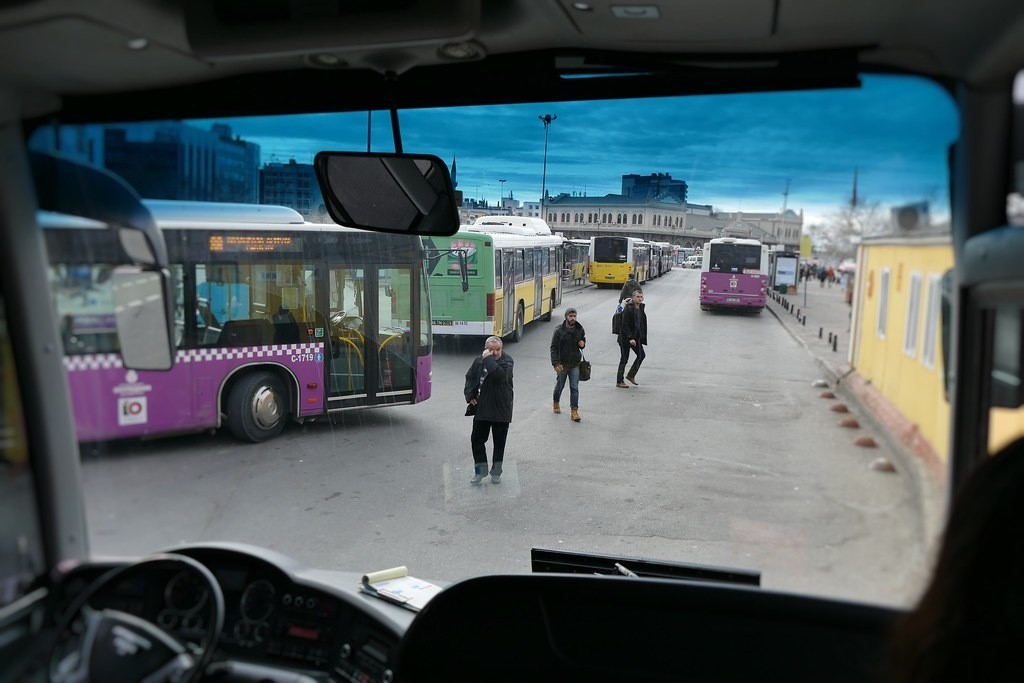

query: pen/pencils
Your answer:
[358,585,420,612]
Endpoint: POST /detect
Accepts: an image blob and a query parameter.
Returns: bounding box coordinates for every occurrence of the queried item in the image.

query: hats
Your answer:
[565,308,577,318]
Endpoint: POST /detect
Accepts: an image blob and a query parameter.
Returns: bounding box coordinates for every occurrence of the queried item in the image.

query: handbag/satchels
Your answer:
[578,349,591,380]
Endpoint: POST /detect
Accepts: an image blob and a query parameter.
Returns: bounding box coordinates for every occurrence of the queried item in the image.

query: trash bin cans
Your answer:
[779,283,787,294]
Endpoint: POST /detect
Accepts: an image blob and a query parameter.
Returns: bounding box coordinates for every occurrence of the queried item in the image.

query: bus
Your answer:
[0,199,470,460]
[384,216,566,344]
[587,233,674,289]
[560,234,591,283]
[698,236,770,315]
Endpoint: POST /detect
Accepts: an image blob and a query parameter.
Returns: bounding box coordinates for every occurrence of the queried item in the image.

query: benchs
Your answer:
[61,297,300,354]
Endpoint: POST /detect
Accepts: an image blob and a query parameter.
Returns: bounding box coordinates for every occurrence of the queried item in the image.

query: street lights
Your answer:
[537,113,559,219]
[498,178,506,207]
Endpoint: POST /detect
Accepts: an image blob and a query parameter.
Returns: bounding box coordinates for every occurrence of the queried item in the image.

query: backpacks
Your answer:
[612,304,632,334]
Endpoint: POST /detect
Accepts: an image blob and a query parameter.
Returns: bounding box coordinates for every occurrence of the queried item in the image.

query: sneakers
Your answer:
[571,409,581,420]
[554,400,560,414]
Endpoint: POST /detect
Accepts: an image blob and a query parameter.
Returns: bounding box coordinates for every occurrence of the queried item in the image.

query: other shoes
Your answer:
[616,381,629,388]
[626,375,638,385]
[492,474,501,483]
[470,473,488,483]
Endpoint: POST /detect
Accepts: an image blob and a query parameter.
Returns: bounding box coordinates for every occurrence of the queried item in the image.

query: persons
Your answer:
[799,263,835,288]
[550,306,586,421]
[465,336,514,484]
[617,273,647,387]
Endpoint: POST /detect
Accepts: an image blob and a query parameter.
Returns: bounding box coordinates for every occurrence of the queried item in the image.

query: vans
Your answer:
[681,256,703,269]
